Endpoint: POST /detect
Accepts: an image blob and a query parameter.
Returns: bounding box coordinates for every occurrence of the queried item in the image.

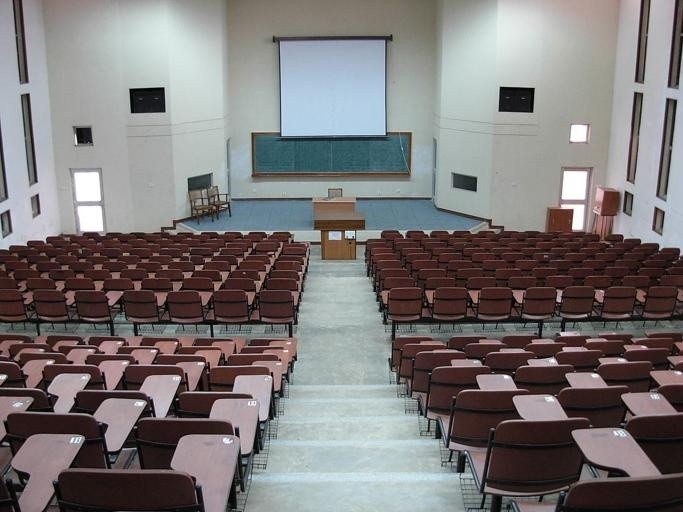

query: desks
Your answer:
[311,195,357,220]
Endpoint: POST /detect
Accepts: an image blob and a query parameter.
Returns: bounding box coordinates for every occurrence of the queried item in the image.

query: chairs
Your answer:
[188,186,215,224]
[206,186,234,220]
[328,188,342,198]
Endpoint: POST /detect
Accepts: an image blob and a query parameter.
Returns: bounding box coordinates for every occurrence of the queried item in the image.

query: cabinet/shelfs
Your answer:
[545,206,574,232]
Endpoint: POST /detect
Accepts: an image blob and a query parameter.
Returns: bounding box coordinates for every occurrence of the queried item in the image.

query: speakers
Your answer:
[498,87,535,113]
[129,87,166,114]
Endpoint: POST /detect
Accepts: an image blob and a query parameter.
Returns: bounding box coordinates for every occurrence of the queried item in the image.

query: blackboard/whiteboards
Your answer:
[251,132,412,178]
